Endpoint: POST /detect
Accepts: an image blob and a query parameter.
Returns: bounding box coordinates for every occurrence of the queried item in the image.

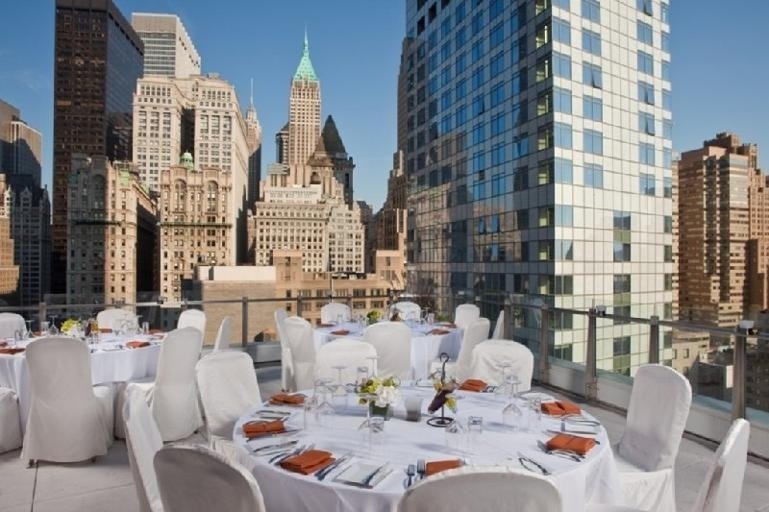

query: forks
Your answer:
[416,459,426,481]
[548,414,603,445]
[406,463,415,491]
[246,407,314,466]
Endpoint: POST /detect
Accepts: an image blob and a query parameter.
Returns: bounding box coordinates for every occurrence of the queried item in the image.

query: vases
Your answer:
[369,401,390,420]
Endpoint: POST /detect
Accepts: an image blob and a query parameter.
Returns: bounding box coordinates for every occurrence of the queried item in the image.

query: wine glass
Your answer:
[304,362,368,430]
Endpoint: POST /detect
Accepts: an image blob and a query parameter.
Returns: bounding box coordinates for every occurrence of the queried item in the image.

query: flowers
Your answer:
[353,375,400,406]
[366,310,383,320]
[60,319,88,336]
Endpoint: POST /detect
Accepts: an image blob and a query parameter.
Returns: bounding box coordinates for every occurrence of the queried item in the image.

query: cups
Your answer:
[497,362,543,438]
[6,314,60,348]
[406,305,435,335]
[404,394,424,422]
[335,312,369,337]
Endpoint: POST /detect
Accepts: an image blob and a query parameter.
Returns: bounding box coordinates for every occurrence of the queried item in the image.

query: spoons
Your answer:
[516,448,550,476]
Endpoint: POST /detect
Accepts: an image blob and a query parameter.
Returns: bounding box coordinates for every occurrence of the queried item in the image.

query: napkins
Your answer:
[243,420,287,438]
[458,379,486,392]
[278,448,338,475]
[2,347,25,355]
[427,329,449,335]
[124,341,152,350]
[547,434,598,458]
[541,401,581,417]
[329,330,351,336]
[270,393,306,408]
[425,459,461,477]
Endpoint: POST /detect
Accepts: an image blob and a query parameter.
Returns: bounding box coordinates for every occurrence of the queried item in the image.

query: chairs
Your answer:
[1,386,22,454]
[454,305,479,330]
[395,466,562,511]
[116,327,205,444]
[176,308,207,356]
[470,339,536,391]
[94,308,138,334]
[285,315,320,390]
[121,388,169,510]
[1,313,28,338]
[198,315,237,419]
[273,305,293,388]
[490,310,506,340]
[154,445,267,512]
[611,364,693,510]
[314,338,378,386]
[388,301,419,322]
[319,302,354,322]
[196,349,264,447]
[690,418,749,511]
[22,336,112,468]
[431,317,492,382]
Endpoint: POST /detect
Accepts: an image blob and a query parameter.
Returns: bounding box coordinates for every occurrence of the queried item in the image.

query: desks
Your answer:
[232,381,619,509]
[6,331,163,390]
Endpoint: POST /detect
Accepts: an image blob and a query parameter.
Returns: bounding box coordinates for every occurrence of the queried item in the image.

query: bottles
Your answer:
[368,415,385,458]
[86,313,151,352]
[467,416,486,455]
